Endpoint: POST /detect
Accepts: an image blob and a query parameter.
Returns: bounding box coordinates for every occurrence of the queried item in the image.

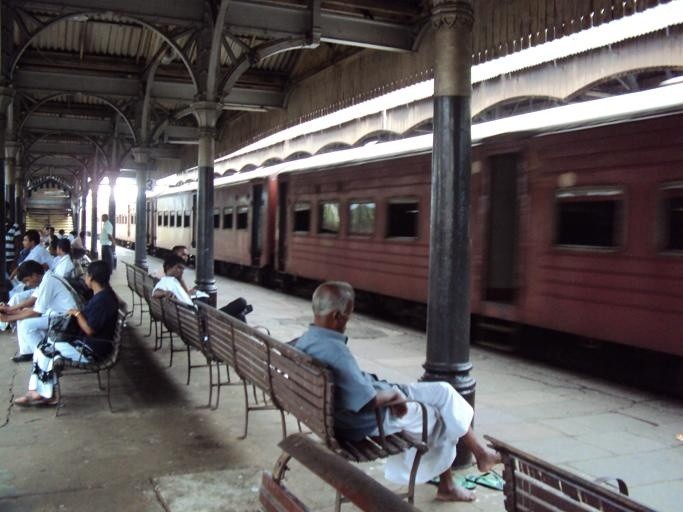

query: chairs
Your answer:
[15,383,60,406]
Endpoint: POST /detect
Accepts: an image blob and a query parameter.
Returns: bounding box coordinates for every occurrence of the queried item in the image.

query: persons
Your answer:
[100,214,115,267]
[152,255,252,323]
[14,259,119,405]
[1,260,80,361]
[293,278,502,501]
[153,245,209,303]
[1,217,92,331]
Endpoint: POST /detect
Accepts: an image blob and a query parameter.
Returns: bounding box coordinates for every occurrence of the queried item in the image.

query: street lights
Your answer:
[47,314,78,341]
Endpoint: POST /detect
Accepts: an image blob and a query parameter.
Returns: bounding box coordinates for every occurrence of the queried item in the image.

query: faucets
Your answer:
[429,470,504,489]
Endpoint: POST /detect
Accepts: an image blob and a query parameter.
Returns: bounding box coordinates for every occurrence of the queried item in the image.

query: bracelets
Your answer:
[75,311,80,316]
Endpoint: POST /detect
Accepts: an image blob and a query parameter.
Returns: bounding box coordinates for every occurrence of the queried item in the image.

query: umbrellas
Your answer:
[10,353,33,362]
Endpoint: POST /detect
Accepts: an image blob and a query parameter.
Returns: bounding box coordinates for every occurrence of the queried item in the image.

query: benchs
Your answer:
[484,434,658,512]
[128,264,260,406]
[40,260,126,417]
[197,301,428,512]
